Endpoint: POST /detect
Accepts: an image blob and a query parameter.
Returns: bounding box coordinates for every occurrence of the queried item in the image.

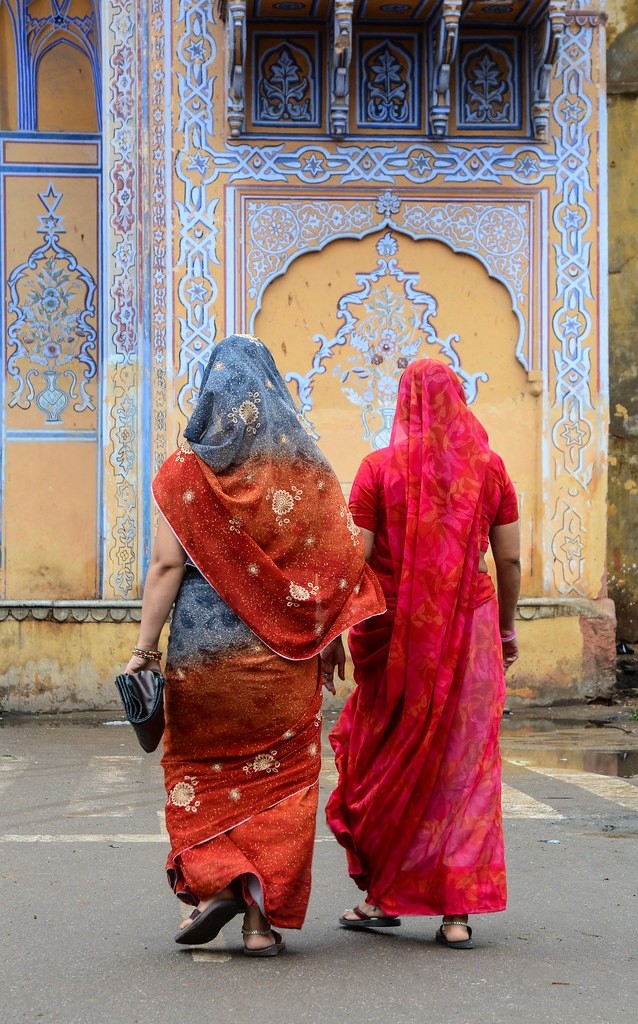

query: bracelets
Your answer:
[131,646,162,662]
[499,628,517,643]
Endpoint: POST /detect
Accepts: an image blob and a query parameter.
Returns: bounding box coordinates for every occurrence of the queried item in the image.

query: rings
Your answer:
[325,673,328,676]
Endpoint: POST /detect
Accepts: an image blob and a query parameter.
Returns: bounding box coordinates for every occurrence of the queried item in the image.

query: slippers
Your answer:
[175,900,239,945]
[435,924,473,948]
[244,929,285,956]
[338,905,401,927]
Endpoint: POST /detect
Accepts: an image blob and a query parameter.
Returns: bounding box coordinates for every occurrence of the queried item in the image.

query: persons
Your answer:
[321,362,522,949]
[122,334,348,957]
